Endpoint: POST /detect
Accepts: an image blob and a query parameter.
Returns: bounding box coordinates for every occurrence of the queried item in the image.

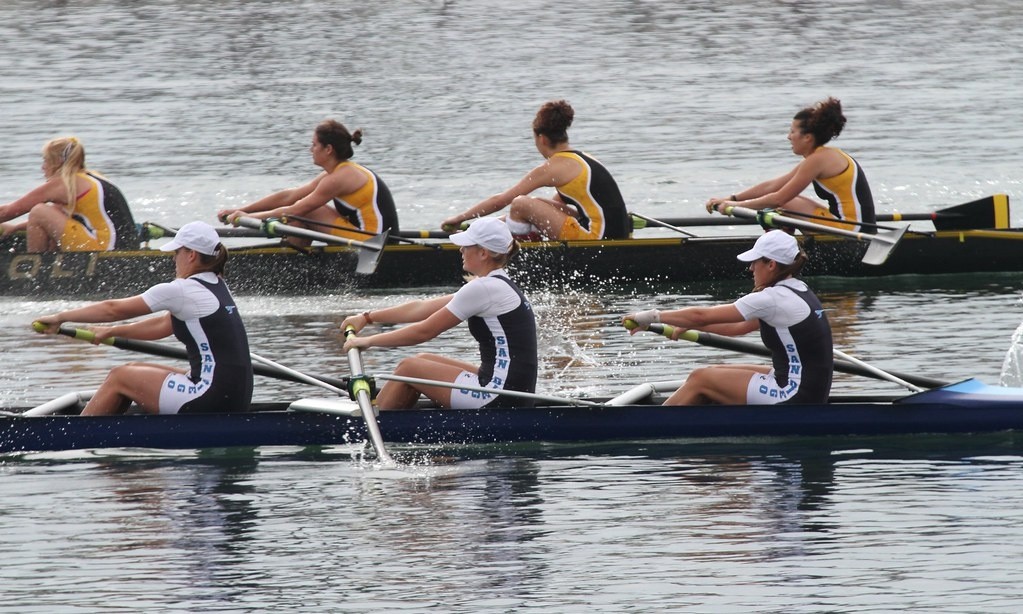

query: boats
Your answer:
[0,382,1022,450]
[0,219,1023,295]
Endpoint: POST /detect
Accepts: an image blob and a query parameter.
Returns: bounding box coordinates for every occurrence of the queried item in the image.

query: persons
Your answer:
[0,138,140,251]
[621,230,834,405]
[32,222,254,415]
[218,120,401,246]
[341,216,537,409]
[706,97,877,236]
[441,102,633,242]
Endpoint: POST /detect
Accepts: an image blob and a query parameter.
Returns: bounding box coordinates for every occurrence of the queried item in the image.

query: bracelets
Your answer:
[731,194,737,200]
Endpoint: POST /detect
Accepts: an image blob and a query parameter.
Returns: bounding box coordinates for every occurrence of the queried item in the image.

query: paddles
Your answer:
[218,212,394,275]
[710,202,912,267]
[13,220,455,240]
[31,318,385,399]
[620,319,952,389]
[441,191,1012,233]
[341,323,407,472]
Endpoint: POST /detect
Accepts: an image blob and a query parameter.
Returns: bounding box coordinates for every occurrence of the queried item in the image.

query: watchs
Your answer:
[362,310,373,324]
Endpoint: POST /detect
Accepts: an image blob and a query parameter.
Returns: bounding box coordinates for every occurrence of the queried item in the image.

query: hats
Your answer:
[160,222,220,256]
[449,216,514,255]
[736,229,800,265]
